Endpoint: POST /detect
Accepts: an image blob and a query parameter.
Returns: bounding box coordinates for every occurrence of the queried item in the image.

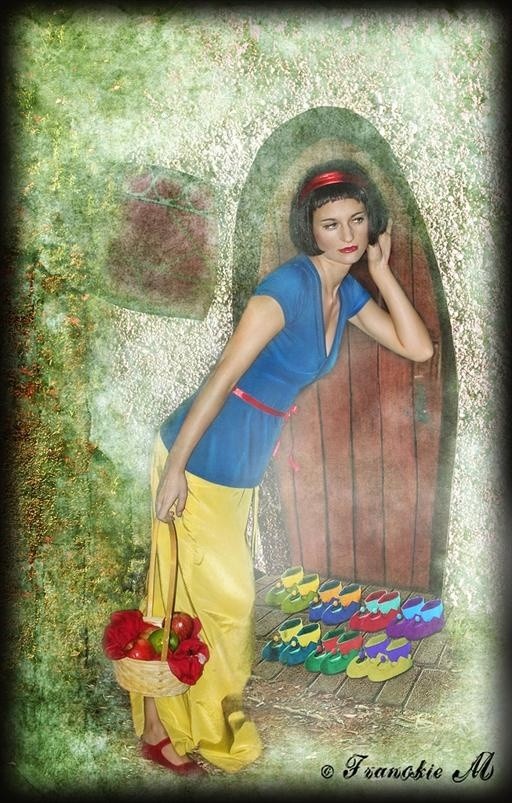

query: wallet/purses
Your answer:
[113,514,191,697]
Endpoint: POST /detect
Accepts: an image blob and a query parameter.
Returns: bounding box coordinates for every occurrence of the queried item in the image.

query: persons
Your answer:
[127,161,437,779]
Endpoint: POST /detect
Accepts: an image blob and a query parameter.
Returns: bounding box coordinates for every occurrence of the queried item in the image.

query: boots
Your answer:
[262,566,444,682]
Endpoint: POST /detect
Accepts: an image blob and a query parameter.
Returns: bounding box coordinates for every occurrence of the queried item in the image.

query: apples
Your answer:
[123,612,192,660]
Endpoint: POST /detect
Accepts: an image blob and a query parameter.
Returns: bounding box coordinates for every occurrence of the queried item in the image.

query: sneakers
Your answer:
[141,738,201,775]
